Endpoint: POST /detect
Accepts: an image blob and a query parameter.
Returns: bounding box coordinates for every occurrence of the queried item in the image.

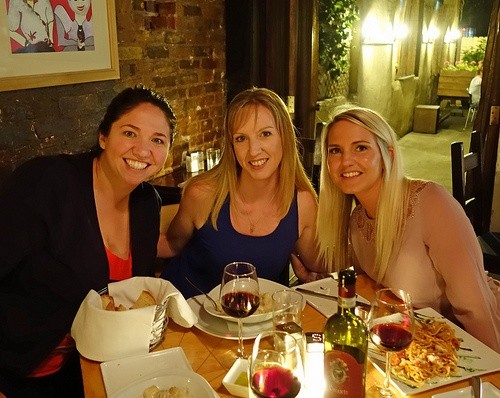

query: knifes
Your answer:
[295,287,370,309]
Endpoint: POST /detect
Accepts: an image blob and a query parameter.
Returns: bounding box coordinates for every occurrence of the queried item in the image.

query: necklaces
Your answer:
[245,208,258,232]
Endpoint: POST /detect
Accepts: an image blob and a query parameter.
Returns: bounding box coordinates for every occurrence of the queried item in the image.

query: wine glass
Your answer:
[369,287,416,398]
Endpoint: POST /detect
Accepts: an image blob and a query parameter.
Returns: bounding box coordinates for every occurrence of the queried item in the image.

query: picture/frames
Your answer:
[0,0,121,92]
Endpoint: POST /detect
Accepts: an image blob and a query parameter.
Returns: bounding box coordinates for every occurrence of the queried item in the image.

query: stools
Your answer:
[414,105,440,134]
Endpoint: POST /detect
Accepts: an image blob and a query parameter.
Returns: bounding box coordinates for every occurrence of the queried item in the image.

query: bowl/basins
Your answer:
[204,278,303,323]
[222,358,250,397]
[431,382,500,398]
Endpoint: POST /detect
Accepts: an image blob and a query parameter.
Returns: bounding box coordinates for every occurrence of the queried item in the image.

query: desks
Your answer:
[148,161,214,205]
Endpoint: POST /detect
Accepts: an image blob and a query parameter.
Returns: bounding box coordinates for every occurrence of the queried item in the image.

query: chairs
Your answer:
[451,129,500,276]
[464,92,479,129]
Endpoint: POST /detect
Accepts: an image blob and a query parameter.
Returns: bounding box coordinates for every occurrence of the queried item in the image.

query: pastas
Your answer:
[390,322,460,382]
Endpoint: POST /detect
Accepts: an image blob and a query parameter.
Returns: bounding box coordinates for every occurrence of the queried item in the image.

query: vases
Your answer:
[437,70,476,97]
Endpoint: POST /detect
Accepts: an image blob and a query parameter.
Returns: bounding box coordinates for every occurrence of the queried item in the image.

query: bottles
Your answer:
[323,268,368,398]
[248,331,301,398]
[187,150,204,173]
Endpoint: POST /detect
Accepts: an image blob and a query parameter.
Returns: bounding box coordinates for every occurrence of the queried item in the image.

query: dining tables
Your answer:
[79,270,500,398]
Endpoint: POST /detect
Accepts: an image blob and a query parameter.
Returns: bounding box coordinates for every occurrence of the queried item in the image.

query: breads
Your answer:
[100,290,156,311]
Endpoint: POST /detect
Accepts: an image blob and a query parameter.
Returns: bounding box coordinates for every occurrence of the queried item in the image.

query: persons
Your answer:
[469,69,483,94]
[290,108,500,354]
[157,88,335,300]
[0,83,177,398]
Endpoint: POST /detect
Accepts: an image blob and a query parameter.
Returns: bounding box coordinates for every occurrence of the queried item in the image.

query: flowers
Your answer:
[442,60,485,72]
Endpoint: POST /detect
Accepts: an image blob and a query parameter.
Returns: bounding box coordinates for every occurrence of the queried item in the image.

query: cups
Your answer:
[206,148,220,171]
[272,290,303,330]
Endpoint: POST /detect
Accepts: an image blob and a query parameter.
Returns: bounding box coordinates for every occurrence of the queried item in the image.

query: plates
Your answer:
[186,294,274,339]
[357,308,500,395]
[292,276,372,320]
[100,347,221,398]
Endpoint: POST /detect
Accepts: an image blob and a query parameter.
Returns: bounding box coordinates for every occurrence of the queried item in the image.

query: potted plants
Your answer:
[316,0,360,101]
